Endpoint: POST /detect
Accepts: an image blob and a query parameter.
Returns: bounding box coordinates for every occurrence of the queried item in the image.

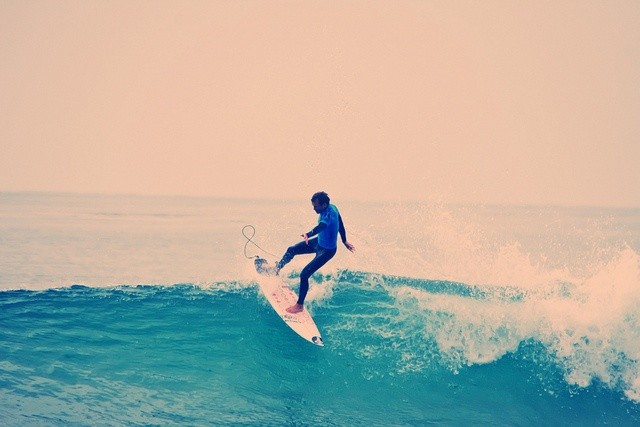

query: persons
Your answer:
[261,191,356,313]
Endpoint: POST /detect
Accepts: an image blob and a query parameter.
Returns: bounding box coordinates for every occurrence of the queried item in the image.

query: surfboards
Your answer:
[254,257,325,347]
